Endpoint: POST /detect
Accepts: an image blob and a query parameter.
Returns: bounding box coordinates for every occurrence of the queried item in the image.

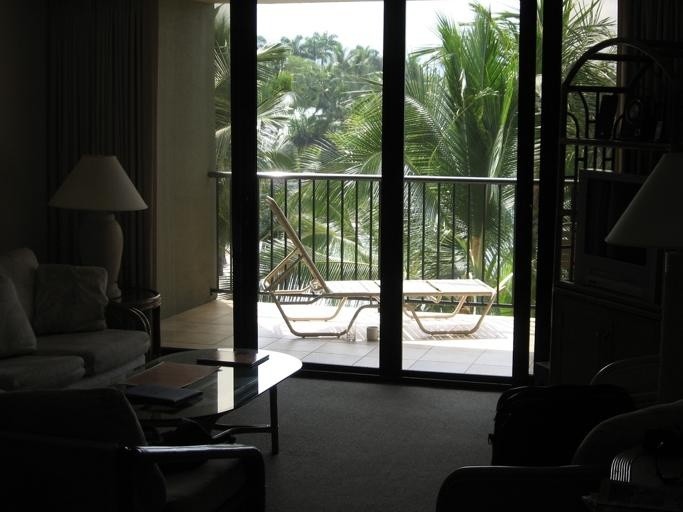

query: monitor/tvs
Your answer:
[574,174,662,305]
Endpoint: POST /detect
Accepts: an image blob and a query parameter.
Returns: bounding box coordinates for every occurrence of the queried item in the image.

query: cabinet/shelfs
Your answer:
[546,282,666,408]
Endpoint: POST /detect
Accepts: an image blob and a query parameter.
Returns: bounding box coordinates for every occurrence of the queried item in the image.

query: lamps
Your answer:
[602,151,682,406]
[48,154,150,301]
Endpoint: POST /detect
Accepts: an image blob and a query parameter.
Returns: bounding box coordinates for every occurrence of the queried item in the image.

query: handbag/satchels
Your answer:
[487,382,637,465]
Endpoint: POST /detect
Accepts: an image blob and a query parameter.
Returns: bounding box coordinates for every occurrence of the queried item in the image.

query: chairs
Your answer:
[1,388,266,511]
[258,194,499,340]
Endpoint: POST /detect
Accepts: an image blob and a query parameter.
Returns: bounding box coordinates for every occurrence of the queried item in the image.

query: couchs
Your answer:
[0,245,152,388]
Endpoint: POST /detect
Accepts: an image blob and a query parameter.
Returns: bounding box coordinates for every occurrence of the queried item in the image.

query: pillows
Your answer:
[0,273,39,357]
[31,263,109,336]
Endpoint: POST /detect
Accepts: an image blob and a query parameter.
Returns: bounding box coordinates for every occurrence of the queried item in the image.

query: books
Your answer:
[122,381,204,405]
[197,349,269,368]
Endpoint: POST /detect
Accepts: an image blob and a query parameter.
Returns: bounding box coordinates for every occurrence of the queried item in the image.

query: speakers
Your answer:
[593,94,618,140]
[620,94,654,142]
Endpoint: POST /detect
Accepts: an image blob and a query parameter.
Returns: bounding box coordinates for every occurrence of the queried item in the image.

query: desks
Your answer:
[110,286,160,350]
